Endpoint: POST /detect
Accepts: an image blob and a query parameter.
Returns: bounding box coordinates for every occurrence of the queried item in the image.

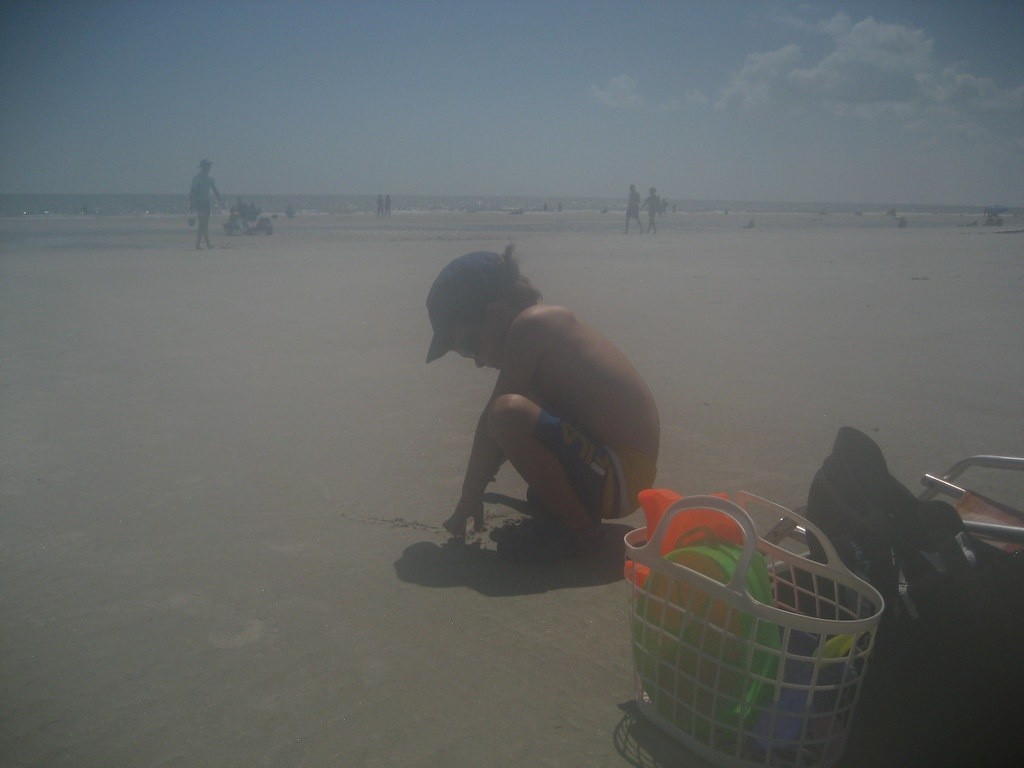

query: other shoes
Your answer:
[496,510,601,561]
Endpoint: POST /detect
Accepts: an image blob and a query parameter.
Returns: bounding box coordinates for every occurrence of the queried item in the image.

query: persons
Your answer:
[640,188,662,233]
[377,193,391,219]
[659,198,668,216]
[624,185,643,233]
[422,253,659,563]
[226,195,275,236]
[189,158,223,250]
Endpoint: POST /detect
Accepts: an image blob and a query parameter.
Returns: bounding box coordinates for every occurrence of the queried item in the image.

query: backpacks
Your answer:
[629,526,784,742]
[805,426,1024,768]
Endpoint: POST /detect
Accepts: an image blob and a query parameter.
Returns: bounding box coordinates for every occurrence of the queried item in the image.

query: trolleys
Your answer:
[641,453,1024,768]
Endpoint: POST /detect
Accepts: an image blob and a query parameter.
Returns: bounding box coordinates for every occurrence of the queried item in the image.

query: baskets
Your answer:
[624,490,884,768]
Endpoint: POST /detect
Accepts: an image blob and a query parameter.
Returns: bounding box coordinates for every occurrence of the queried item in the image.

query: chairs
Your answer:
[755,452,1024,767]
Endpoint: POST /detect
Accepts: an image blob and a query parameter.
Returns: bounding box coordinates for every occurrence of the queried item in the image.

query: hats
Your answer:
[425,253,500,364]
[200,160,213,169]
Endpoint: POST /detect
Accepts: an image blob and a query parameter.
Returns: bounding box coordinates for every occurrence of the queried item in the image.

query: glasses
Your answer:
[455,306,492,360]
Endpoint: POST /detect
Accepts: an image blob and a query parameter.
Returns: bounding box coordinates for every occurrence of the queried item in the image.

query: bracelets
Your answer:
[465,465,497,483]
